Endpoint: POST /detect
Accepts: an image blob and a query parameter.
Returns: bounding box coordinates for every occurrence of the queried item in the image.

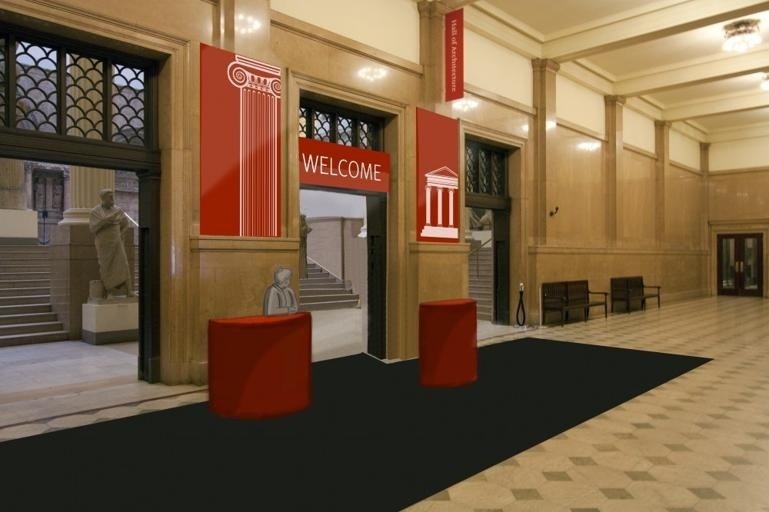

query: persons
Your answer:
[262,264,299,318]
[86,187,139,301]
